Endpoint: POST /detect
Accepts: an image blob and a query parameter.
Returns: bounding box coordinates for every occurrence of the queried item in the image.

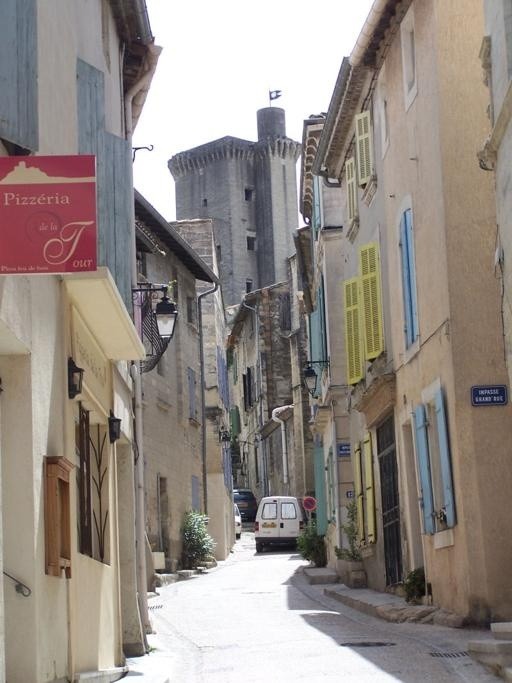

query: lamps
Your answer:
[108,408,122,443]
[132,284,180,343]
[67,355,85,400]
[301,355,331,400]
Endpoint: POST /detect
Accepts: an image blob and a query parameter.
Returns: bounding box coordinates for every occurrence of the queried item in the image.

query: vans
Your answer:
[254,495,307,553]
[233,488,258,520]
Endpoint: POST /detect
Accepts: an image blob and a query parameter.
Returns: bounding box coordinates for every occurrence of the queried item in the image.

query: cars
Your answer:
[234,503,242,538]
